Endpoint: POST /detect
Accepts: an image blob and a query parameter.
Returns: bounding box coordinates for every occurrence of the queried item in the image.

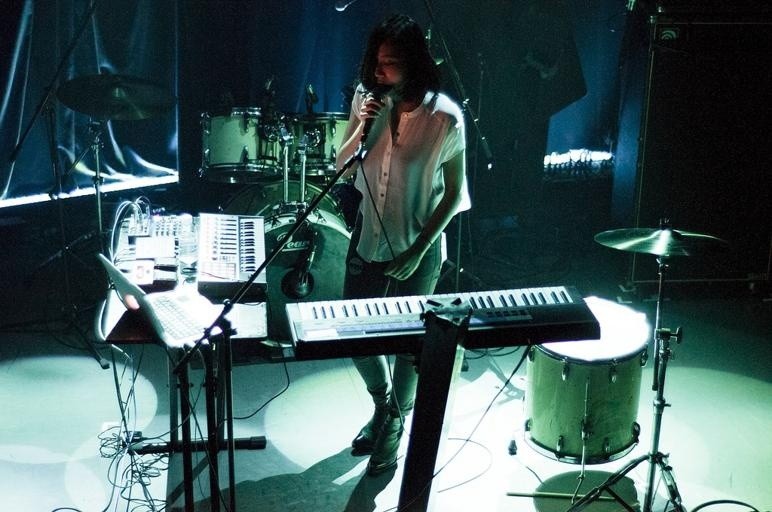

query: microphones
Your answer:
[363,82,389,143]
[308,85,319,104]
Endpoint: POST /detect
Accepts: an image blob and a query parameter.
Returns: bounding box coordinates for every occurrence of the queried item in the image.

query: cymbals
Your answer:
[594,227,726,257]
[57,73,179,121]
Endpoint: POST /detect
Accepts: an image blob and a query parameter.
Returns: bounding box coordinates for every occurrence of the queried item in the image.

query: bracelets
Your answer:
[420,234,433,246]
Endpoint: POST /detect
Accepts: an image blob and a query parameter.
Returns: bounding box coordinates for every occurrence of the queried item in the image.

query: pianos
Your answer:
[196,212,269,297]
[285,284,601,362]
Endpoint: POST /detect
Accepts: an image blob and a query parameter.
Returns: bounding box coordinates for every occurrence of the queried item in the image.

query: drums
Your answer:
[198,107,285,186]
[220,181,354,302]
[290,113,357,178]
[526,291,654,466]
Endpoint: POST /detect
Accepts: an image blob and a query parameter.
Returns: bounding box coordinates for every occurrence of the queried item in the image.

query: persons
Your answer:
[336,12,473,477]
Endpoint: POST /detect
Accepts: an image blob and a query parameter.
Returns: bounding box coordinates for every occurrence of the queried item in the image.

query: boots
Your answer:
[367,412,405,474]
[352,389,391,452]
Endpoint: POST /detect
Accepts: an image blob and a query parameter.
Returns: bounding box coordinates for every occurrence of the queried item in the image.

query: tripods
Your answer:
[424,0,494,372]
[567,257,683,512]
[436,52,526,286]
[1,1,107,368]
